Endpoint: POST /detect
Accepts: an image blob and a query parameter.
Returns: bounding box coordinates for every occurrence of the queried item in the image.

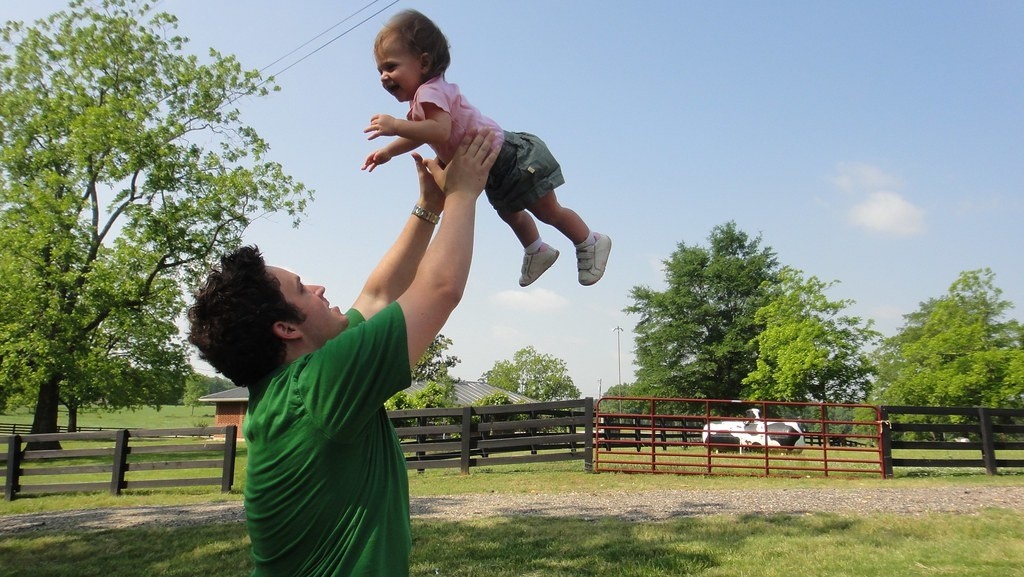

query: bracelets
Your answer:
[412,206,440,225]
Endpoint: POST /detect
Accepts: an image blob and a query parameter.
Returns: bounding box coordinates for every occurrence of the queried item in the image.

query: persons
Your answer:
[186,124,499,577]
[361,9,612,287]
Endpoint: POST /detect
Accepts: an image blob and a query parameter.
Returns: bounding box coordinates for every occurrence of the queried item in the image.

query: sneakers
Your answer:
[575,234,612,286]
[519,242,560,287]
